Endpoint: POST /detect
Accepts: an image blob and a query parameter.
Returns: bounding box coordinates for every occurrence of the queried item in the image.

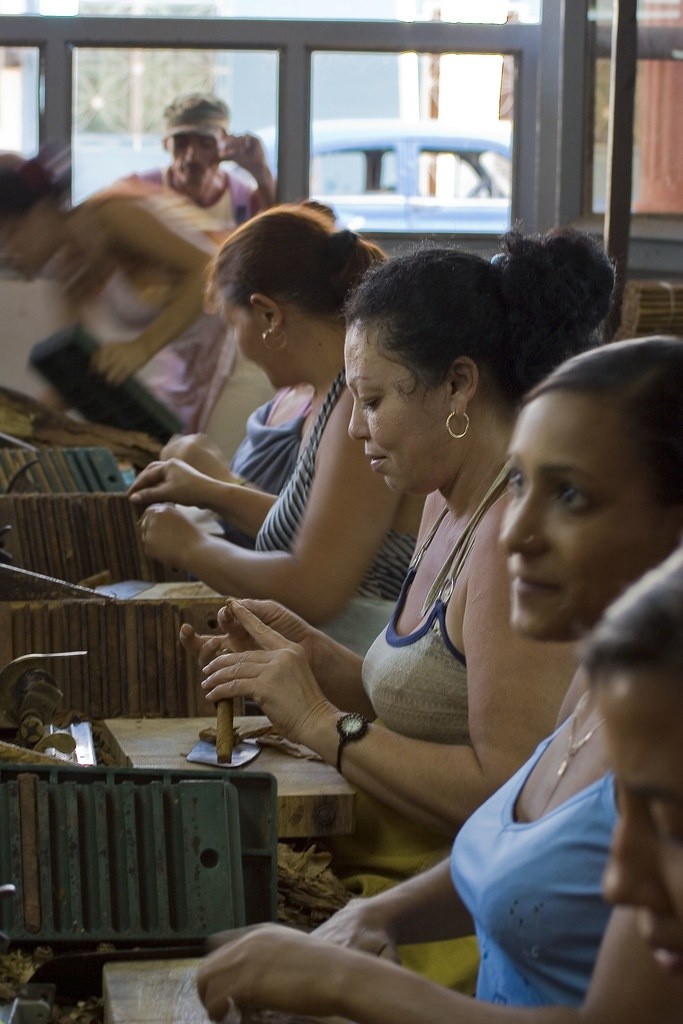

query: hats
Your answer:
[584,549,683,651]
[161,91,233,151]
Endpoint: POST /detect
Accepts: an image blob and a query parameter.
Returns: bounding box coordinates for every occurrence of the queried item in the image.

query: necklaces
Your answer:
[539,718,604,819]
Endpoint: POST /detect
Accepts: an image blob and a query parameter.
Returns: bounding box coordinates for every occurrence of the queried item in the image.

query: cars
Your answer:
[308,117,606,231]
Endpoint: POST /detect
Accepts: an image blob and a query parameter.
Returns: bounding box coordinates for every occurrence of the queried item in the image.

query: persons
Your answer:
[128,205,424,627]
[197,335,683,1024]
[179,230,616,998]
[0,90,336,466]
[581,547,683,980]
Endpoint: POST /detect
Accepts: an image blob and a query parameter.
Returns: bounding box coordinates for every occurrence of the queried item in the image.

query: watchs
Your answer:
[337,713,368,773]
